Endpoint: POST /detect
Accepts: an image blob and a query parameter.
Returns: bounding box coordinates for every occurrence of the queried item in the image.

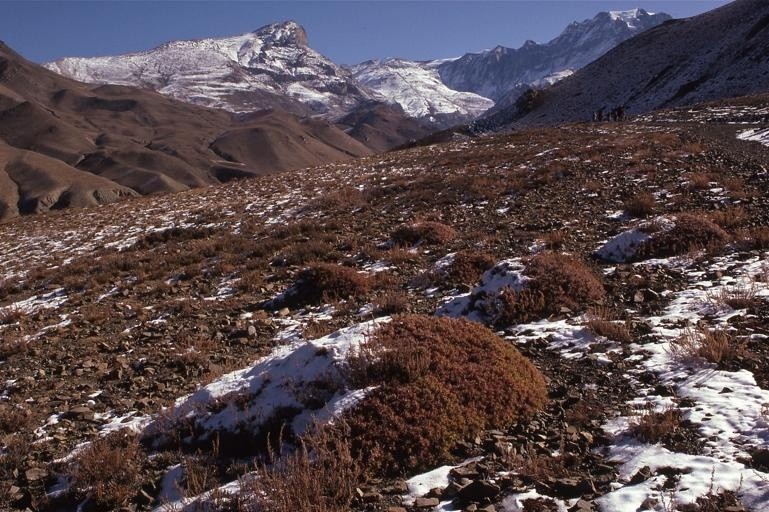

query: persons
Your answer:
[592,103,625,121]
